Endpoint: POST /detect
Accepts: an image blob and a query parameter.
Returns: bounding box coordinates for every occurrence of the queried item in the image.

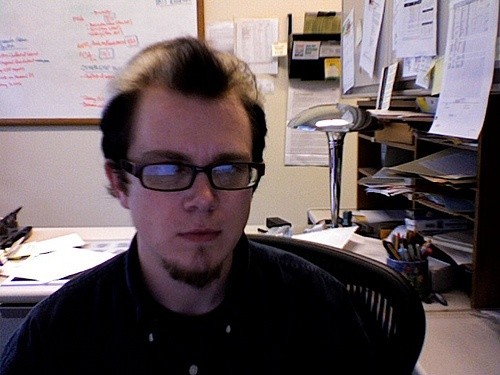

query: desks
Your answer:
[0,226,500,375]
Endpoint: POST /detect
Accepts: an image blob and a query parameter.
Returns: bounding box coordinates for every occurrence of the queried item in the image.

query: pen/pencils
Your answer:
[381,230,433,261]
[257,227,267,233]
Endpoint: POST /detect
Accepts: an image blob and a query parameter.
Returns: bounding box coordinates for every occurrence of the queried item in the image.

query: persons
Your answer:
[0,37,369,375]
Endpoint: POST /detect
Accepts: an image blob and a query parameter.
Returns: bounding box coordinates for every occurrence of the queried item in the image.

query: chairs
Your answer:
[246,234,426,375]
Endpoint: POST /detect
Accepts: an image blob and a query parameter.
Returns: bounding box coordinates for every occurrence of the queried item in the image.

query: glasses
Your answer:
[117,158,266,192]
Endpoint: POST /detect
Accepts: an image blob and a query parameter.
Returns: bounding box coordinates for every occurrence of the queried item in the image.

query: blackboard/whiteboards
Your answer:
[339,0,500,98]
[0,0,205,127]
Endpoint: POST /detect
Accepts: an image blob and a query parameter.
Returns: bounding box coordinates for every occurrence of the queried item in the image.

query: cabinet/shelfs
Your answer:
[356,92,500,310]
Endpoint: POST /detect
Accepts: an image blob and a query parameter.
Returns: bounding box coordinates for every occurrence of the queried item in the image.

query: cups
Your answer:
[387,253,429,298]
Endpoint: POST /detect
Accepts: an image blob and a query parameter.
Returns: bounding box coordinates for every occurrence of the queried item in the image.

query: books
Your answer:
[423,231,475,253]
